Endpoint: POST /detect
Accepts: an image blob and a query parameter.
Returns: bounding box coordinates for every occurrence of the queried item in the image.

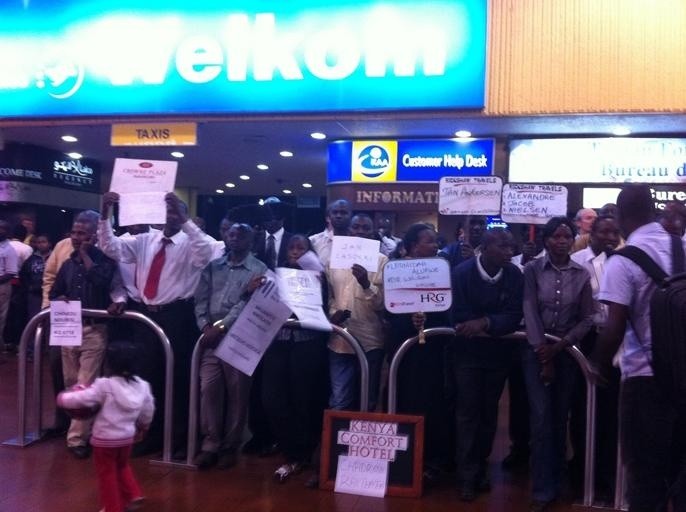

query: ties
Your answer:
[143,239,172,298]
[264,236,276,269]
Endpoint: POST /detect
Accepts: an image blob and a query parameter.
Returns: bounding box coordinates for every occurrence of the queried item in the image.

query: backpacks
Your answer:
[621,239,686,398]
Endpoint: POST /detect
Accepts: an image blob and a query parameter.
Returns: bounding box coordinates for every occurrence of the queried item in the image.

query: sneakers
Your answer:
[458,467,491,501]
[197,452,217,469]
[132,436,162,456]
[70,446,92,458]
[172,441,187,460]
[45,427,62,437]
[508,470,529,485]
[0,343,37,362]
[222,449,237,467]
[529,497,554,512]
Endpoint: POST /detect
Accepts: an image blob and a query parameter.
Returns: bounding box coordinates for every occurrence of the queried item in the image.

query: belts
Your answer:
[145,305,163,313]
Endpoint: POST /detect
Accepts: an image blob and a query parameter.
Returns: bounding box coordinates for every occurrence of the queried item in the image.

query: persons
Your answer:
[2,182,686,512]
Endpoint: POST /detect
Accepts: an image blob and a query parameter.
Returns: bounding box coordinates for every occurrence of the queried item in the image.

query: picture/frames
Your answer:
[319,409,423,497]
